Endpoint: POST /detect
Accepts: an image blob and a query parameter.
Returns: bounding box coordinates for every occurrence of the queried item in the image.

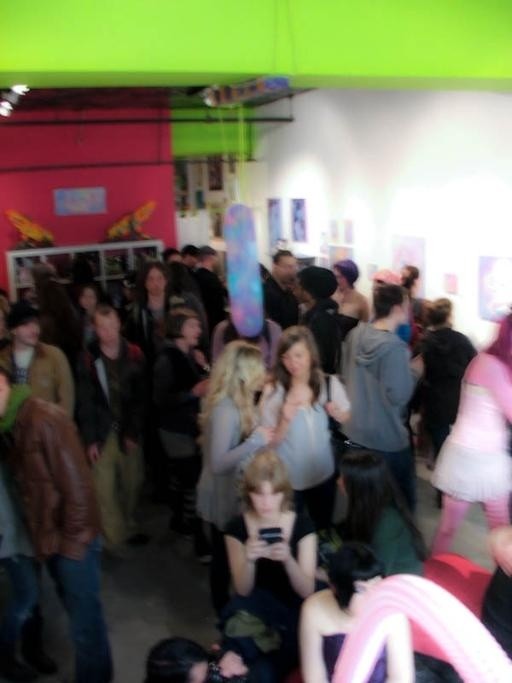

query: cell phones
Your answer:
[258,528,282,544]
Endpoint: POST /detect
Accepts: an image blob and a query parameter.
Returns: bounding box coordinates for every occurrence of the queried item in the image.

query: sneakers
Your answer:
[191,533,212,565]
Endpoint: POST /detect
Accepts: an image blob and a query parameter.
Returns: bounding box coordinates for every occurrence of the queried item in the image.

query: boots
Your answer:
[0,641,38,682]
[18,618,58,675]
